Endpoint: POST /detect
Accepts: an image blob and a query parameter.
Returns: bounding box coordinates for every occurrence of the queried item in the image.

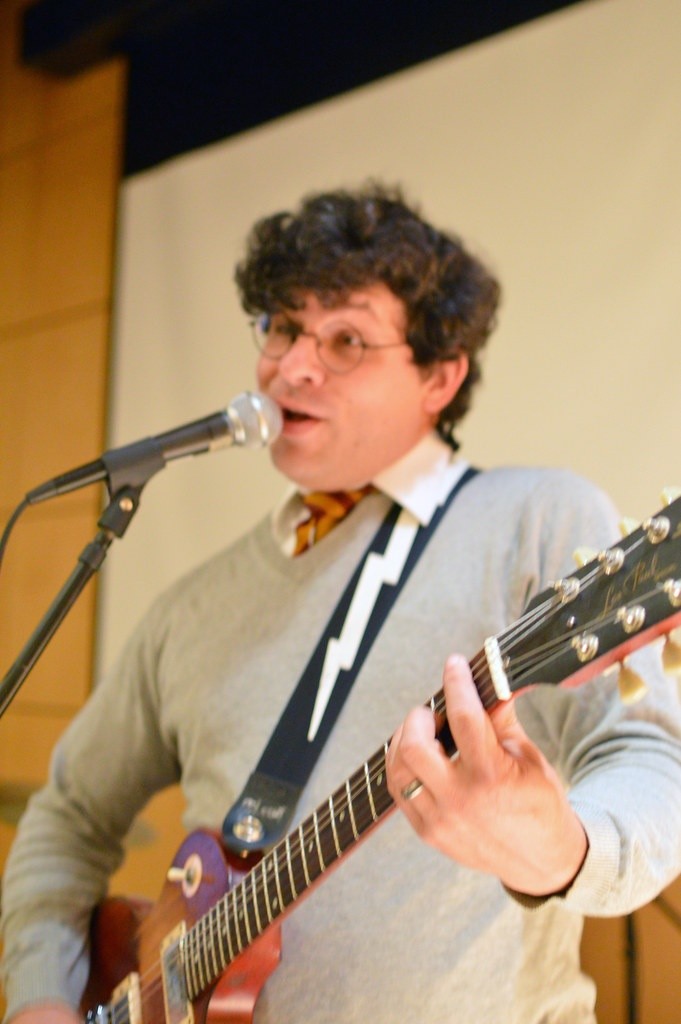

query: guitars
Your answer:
[87,488,681,1024]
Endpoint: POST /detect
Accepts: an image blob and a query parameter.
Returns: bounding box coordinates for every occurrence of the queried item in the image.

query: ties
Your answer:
[294,486,377,553]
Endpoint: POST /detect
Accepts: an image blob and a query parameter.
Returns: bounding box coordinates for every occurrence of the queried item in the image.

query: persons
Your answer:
[0,190,680,1024]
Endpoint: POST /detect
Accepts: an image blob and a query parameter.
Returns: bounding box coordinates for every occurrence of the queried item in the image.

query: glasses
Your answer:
[250,310,411,374]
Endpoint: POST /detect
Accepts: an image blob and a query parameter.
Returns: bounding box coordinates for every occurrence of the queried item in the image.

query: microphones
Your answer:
[26,390,285,505]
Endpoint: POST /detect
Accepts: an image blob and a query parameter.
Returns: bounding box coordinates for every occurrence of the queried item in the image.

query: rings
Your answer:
[399,777,426,804]
